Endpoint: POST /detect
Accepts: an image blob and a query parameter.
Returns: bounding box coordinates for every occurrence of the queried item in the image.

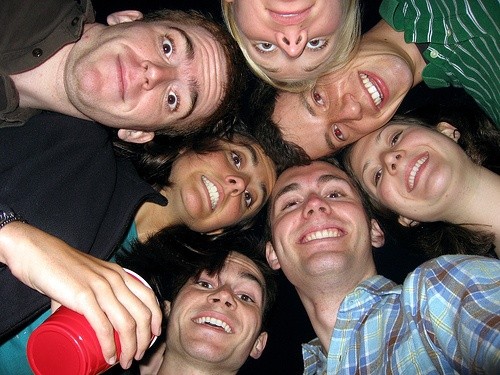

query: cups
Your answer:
[27,268,159,375]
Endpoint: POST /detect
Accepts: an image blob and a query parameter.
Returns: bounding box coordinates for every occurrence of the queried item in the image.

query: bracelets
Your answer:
[0,208,25,229]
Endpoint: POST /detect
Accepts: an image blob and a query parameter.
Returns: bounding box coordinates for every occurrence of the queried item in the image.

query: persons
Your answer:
[257,157,500,375]
[333,114,500,260]
[247,0,500,166]
[0,0,244,144]
[221,0,361,92]
[156,231,279,375]
[0,108,313,375]
